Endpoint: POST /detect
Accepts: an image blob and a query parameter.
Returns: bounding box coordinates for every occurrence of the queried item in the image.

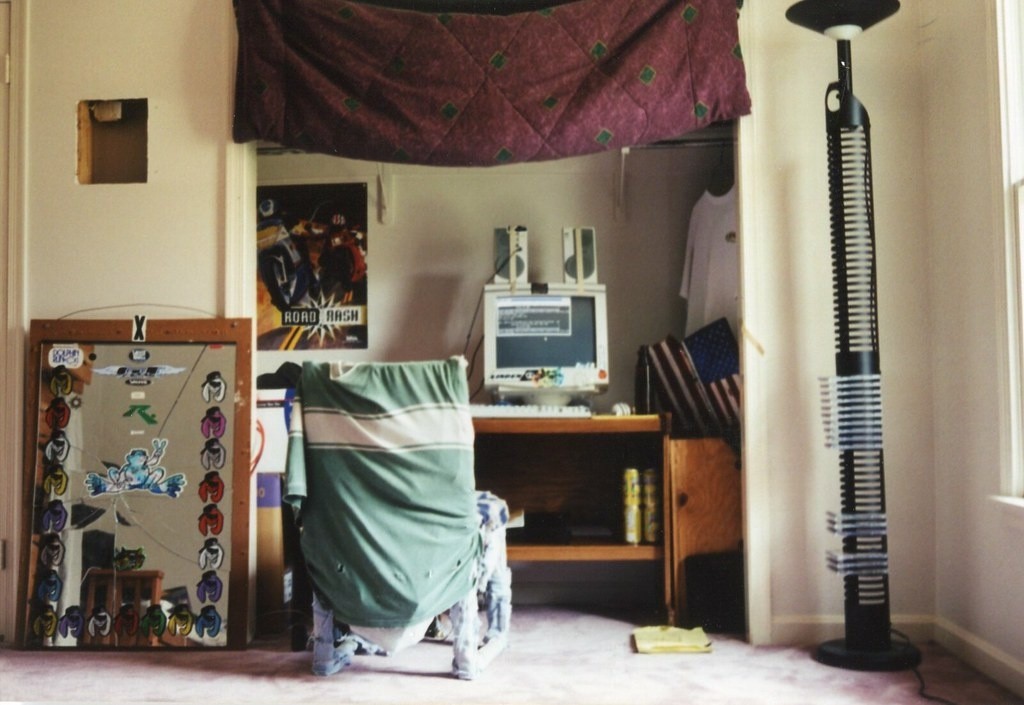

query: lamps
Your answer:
[784,0,927,670]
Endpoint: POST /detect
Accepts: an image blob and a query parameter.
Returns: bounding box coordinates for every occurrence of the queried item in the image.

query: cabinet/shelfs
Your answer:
[671,439,744,624]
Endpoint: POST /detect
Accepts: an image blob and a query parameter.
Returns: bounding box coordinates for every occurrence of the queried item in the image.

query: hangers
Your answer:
[705,141,733,193]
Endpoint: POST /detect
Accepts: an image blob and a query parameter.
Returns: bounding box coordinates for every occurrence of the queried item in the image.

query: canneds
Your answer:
[622,468,661,544]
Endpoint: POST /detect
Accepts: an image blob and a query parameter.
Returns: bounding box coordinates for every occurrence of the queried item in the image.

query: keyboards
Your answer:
[470,403,592,419]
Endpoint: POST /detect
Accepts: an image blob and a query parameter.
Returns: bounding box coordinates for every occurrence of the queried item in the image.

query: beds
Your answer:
[140,600,226,647]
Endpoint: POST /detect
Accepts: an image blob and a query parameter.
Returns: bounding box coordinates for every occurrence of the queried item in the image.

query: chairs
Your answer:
[296,357,512,680]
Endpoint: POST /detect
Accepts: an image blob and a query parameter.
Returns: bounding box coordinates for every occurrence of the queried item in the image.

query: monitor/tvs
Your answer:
[483,289,610,406]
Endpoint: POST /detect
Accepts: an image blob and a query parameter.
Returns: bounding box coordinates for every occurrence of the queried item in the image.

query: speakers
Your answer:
[563,225,598,286]
[491,228,528,287]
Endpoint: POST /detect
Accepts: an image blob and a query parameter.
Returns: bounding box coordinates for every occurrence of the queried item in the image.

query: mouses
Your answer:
[611,402,632,417]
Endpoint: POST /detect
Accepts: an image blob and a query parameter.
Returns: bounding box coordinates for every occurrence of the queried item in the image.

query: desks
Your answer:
[83,568,163,647]
[474,414,670,564]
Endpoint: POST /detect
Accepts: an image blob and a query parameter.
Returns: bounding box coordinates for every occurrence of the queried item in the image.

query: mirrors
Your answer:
[14,316,253,654]
